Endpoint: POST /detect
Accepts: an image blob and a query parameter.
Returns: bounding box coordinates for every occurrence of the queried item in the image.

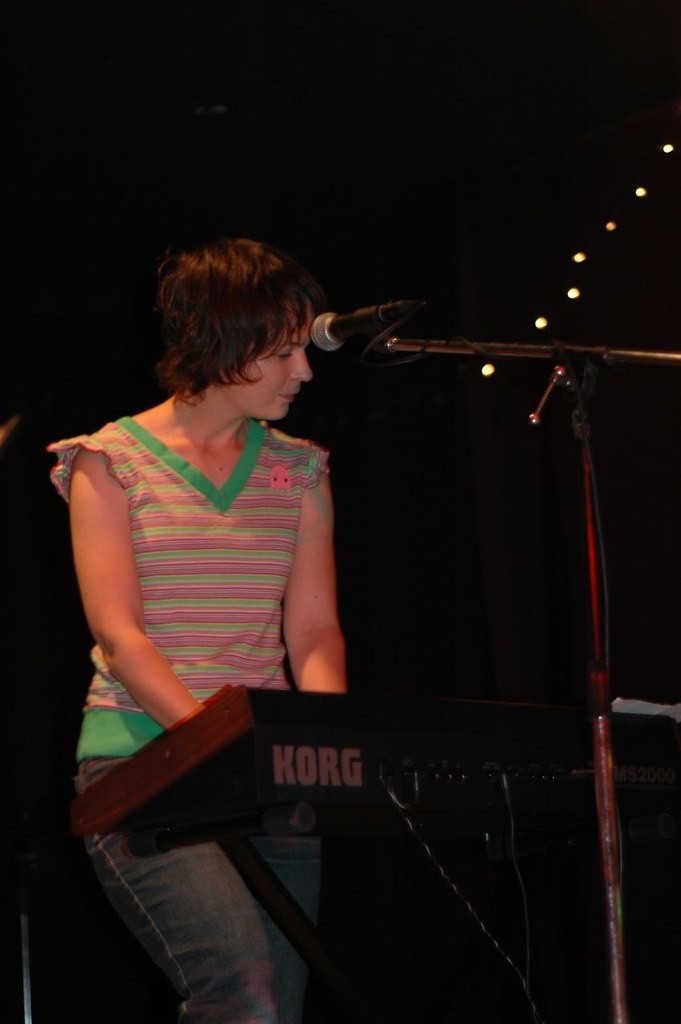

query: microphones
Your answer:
[309,297,428,352]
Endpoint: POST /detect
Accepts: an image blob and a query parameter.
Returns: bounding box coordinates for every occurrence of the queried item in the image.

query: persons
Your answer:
[46,238,351,1024]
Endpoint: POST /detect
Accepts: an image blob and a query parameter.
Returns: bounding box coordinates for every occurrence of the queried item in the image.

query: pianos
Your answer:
[73,685,681,860]
